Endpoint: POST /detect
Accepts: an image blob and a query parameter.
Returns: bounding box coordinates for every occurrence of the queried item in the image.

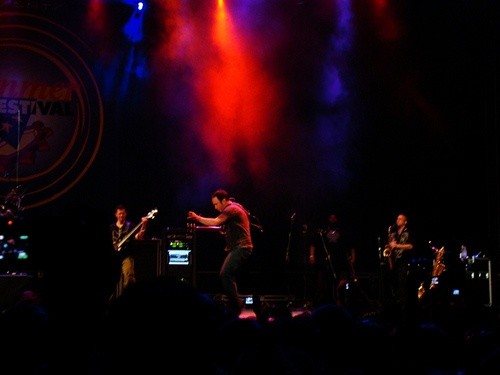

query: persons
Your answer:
[226,196,262,231]
[110,205,149,283]
[383,215,415,293]
[308,211,356,285]
[187,189,254,297]
[0,261,500,375]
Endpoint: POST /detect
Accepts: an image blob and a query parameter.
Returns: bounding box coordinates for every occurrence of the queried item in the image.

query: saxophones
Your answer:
[428,241,447,289]
[383,223,396,270]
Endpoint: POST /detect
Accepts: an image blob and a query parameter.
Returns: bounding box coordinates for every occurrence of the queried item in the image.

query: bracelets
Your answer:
[140,228,146,232]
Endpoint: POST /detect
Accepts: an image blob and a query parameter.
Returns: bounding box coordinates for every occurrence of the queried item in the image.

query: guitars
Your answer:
[112,208,159,253]
